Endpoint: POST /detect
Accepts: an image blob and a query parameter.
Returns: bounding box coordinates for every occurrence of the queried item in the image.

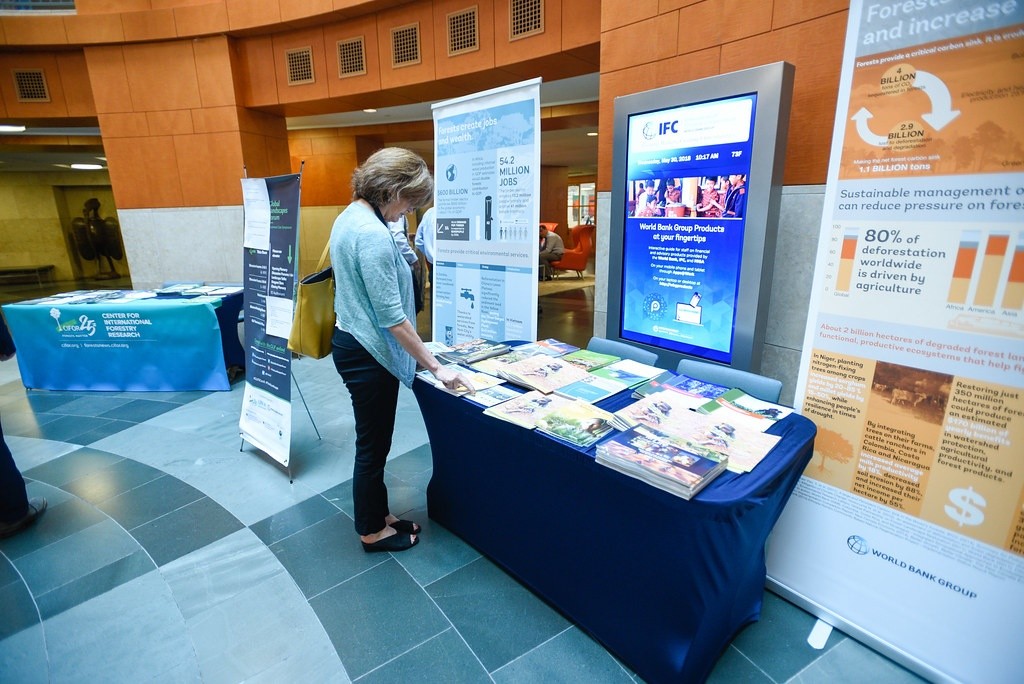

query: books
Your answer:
[182,286,224,295]
[415,334,801,501]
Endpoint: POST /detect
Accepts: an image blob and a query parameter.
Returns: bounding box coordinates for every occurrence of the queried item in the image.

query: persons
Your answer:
[387,213,421,276]
[0,314,48,539]
[635,174,745,219]
[552,418,612,443]
[326,147,476,553]
[539,225,565,281]
[414,205,433,303]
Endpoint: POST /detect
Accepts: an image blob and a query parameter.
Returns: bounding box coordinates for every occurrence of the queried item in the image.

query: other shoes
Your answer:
[361,529,419,553]
[389,519,421,534]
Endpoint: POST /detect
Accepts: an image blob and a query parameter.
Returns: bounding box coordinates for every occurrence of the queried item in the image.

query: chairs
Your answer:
[550,223,593,281]
[539,222,559,250]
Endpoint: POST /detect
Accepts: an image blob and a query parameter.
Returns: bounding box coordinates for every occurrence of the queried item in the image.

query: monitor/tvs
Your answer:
[629,91,757,367]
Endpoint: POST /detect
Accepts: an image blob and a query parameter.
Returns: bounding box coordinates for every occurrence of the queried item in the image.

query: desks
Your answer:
[0,264,52,294]
[2,290,245,392]
[412,342,819,684]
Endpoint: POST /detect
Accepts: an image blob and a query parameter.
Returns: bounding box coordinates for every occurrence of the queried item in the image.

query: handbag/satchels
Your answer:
[287,238,336,360]
